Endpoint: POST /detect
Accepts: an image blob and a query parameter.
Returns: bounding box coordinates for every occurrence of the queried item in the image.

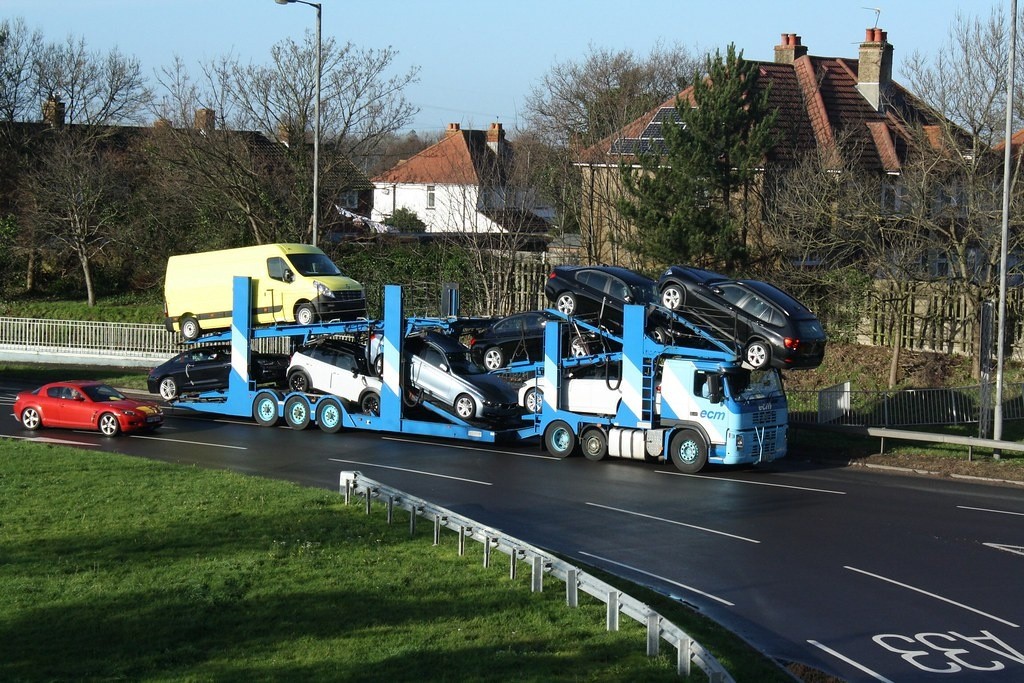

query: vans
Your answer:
[162,242,367,342]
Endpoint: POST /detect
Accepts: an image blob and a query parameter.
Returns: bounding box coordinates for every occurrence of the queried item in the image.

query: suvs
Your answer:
[658,263,827,371]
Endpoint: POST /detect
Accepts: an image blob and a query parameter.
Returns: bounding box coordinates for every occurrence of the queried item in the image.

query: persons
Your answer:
[66,387,80,399]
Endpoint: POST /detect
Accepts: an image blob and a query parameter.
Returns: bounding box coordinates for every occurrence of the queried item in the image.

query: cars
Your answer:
[364,327,519,423]
[517,362,661,418]
[469,310,622,374]
[147,344,292,402]
[545,265,696,346]
[13,379,165,438]
[285,337,383,417]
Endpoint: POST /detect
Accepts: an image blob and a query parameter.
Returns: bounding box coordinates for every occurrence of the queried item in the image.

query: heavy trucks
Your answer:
[163,271,792,476]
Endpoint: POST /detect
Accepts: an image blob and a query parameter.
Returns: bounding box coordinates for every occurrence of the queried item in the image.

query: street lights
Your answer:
[277,0,322,246]
